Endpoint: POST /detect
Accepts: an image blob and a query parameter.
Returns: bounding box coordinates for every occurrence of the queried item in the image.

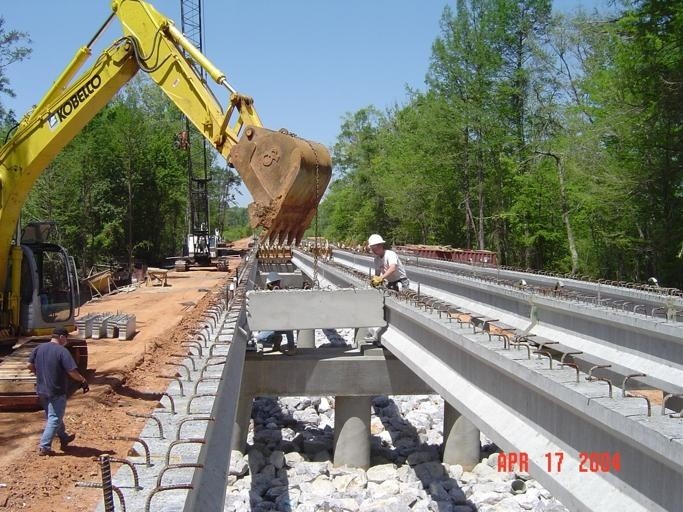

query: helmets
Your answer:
[367,234,385,248]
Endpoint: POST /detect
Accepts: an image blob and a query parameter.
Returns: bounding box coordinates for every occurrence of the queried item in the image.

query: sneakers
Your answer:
[59,433,75,449]
[38,449,56,456]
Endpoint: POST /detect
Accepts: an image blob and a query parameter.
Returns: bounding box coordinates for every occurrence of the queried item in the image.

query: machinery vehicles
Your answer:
[0,1,334,416]
[167,1,232,271]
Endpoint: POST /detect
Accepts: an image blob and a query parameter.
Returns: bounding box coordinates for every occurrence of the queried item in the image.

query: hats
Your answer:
[53,328,70,336]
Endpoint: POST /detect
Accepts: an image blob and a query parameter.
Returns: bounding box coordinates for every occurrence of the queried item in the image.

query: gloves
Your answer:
[371,276,384,288]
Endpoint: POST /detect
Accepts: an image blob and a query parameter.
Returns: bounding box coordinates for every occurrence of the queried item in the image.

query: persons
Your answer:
[366,234,410,291]
[25,326,89,456]
